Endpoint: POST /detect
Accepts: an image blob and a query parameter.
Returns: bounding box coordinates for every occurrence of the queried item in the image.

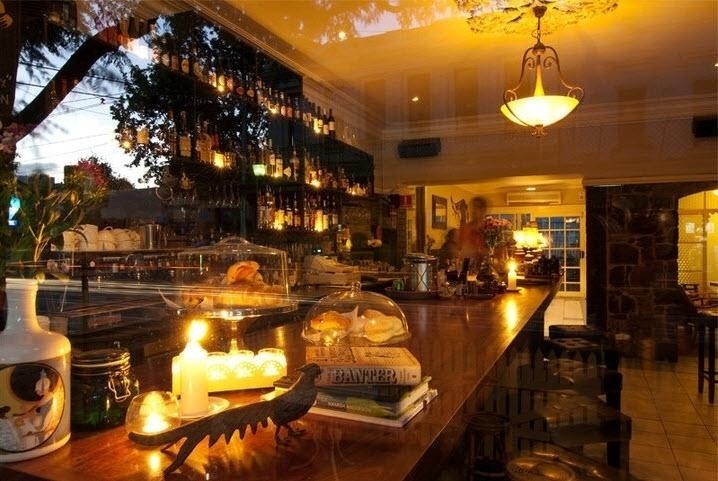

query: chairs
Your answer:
[681,283,703,307]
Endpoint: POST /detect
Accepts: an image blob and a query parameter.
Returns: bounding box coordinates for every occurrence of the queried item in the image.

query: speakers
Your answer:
[692,116,718,138]
[398,137,444,159]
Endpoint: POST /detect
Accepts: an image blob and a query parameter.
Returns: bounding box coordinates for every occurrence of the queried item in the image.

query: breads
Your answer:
[182,261,285,307]
[303,309,406,346]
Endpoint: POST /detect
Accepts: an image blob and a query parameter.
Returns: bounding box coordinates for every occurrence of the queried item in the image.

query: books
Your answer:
[259,344,439,430]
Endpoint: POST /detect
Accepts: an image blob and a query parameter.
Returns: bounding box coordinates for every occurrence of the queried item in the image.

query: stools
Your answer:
[697,307,718,403]
[473,322,642,481]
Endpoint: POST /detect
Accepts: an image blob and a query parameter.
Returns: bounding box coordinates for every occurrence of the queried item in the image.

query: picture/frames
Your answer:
[432,195,447,228]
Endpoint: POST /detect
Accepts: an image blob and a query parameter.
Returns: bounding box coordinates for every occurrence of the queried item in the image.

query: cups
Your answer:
[170,356,181,395]
[257,347,288,388]
[227,349,257,389]
[208,351,227,391]
[168,355,210,418]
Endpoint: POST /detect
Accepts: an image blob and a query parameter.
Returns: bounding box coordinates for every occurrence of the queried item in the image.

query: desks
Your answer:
[0,274,564,481]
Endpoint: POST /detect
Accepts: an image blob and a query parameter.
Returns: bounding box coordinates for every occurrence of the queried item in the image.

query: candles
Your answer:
[505,265,518,292]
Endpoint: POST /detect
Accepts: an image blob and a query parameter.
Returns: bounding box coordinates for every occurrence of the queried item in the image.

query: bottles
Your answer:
[124,388,182,438]
[71,343,141,433]
[0,1,374,279]
[0,275,74,463]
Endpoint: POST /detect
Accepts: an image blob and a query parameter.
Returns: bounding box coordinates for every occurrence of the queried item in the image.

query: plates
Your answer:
[162,395,231,423]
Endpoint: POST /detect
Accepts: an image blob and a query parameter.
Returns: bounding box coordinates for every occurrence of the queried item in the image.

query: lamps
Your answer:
[213,151,235,169]
[706,223,713,232]
[252,164,265,177]
[457,0,620,137]
[685,223,695,233]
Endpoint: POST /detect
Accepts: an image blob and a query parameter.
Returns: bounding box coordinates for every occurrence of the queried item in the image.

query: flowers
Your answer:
[0,156,111,266]
[478,216,516,249]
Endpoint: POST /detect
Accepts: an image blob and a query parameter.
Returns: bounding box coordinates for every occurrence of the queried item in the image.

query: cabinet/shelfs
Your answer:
[175,99,374,260]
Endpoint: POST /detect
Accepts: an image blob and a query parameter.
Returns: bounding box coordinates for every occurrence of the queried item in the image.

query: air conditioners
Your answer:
[507,190,561,205]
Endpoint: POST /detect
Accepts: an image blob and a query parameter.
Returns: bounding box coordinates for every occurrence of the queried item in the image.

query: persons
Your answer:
[459,198,489,256]
[438,228,462,263]
